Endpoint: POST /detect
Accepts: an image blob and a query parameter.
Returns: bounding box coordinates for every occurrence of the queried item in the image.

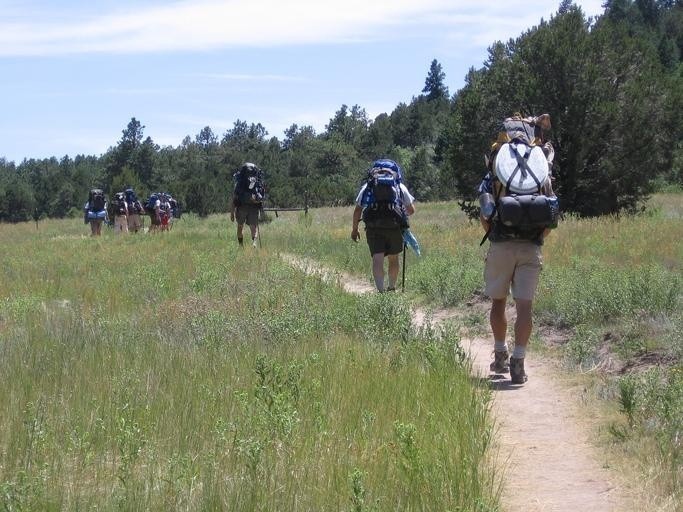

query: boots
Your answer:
[508,356,530,383]
[487,351,510,375]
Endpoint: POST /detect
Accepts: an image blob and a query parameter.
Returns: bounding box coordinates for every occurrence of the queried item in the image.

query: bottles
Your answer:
[479,192,498,221]
[547,194,559,225]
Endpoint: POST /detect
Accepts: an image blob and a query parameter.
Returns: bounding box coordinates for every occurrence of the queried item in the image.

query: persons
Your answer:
[474,149,558,385]
[165,193,174,232]
[125,197,145,233]
[157,191,169,230]
[110,199,128,236]
[349,179,415,295]
[143,198,160,234]
[83,196,107,236]
[230,180,261,250]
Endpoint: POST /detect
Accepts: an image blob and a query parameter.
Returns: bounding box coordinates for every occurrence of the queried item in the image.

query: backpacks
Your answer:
[360,158,405,230]
[82,189,180,220]
[236,162,264,207]
[486,112,560,240]
